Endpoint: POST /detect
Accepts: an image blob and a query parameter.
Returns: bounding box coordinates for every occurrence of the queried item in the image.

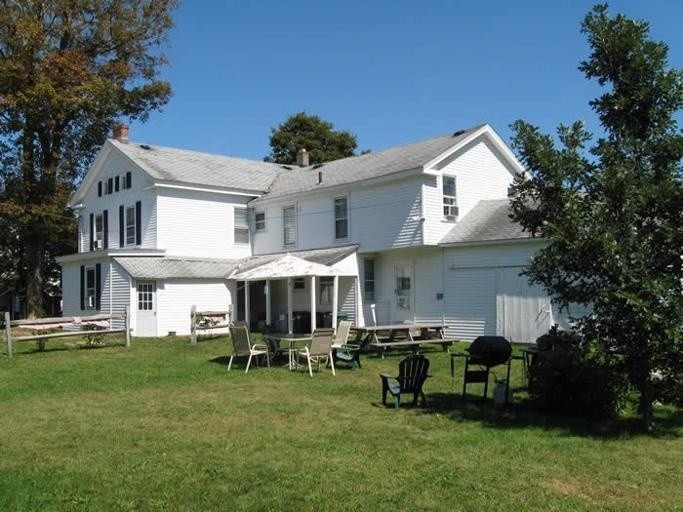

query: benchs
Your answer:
[348,335,461,360]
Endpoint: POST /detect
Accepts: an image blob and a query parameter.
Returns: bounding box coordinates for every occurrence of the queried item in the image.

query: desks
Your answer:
[350,324,451,358]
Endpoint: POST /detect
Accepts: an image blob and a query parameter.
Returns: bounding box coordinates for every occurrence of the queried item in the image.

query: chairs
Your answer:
[379,353,430,409]
[227,320,373,378]
